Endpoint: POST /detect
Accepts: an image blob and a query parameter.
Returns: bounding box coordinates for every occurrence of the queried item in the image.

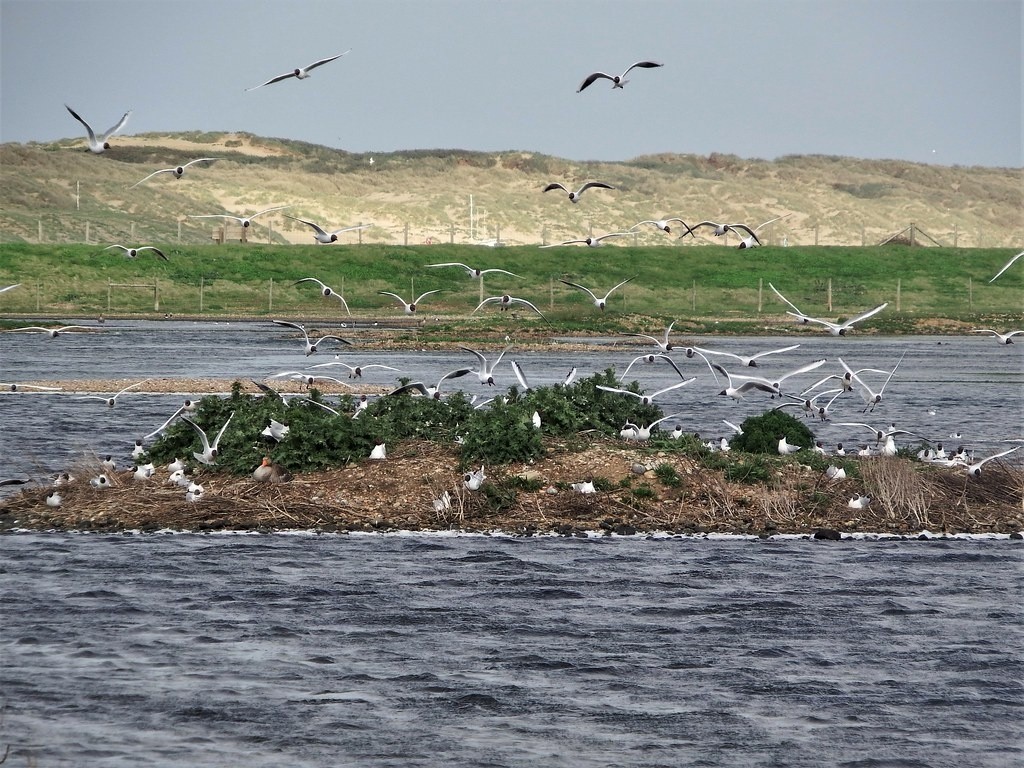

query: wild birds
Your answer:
[575,61,665,94]
[630,218,696,239]
[285,214,374,244]
[101,243,170,266]
[4,324,97,338]
[542,182,616,203]
[128,157,225,190]
[0,383,63,392]
[188,205,288,227]
[244,47,352,92]
[73,377,152,406]
[989,249,1024,284]
[678,220,744,244]
[968,329,1024,346]
[727,213,793,250]
[64,104,133,155]
[45,258,1021,509]
[538,232,643,250]
